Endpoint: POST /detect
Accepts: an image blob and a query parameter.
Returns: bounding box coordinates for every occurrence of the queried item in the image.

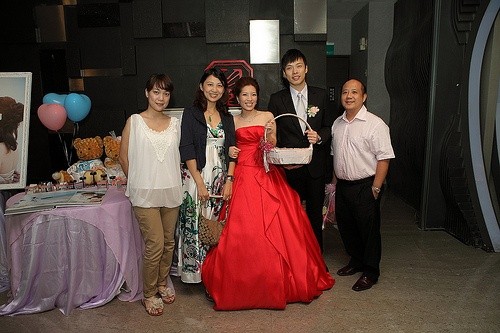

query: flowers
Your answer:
[305,103,320,118]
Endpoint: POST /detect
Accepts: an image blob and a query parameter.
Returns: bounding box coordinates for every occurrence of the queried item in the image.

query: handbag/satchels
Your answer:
[199,194,229,246]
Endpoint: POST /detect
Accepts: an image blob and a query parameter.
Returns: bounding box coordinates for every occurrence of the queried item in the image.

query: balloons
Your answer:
[38,104,66,130]
[64,92,91,122]
[42,93,70,108]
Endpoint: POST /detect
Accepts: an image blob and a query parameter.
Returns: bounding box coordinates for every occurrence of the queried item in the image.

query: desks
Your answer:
[5,185,144,306]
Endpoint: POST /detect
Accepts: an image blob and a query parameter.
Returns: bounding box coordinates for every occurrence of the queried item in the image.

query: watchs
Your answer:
[373,185,381,192]
[227,174,235,181]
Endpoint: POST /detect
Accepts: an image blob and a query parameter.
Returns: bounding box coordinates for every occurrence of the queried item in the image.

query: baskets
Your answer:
[264,113,313,165]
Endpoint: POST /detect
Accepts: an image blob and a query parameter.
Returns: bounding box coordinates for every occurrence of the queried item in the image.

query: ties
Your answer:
[296,94,307,135]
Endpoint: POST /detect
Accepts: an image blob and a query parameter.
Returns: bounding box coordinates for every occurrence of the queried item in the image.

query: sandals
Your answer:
[158,285,176,305]
[141,296,164,316]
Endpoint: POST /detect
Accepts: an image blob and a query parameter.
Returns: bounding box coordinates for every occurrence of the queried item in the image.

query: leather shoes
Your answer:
[352,274,374,291]
[337,264,359,276]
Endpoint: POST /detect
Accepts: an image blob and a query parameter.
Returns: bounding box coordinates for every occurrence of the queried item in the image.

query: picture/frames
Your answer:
[0,70,34,191]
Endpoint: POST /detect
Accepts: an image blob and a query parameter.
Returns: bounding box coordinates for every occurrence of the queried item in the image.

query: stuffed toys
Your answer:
[50,135,106,182]
[99,131,130,185]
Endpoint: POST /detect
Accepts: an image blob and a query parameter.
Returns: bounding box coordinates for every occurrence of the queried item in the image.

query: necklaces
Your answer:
[204,107,217,122]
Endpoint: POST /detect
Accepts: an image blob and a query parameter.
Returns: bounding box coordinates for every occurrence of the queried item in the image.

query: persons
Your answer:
[202,76,336,311]
[268,47,334,273]
[330,78,396,291]
[119,71,184,315]
[169,66,238,283]
[0,96,25,185]
